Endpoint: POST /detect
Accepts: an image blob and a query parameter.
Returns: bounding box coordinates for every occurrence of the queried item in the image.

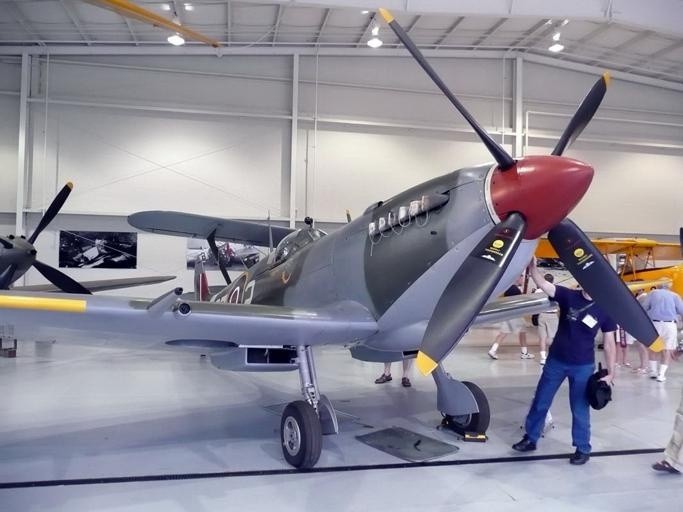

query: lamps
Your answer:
[366,17,384,51]
[548,31,565,53]
[164,17,187,48]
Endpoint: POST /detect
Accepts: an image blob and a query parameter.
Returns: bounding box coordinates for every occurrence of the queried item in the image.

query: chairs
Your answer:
[614,255,632,275]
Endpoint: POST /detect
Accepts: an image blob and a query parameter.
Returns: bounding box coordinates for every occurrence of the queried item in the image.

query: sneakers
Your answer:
[375,373,392,383]
[571,450,591,465]
[488,346,546,365]
[658,376,666,382]
[650,371,658,377]
[512,433,535,451]
[402,377,411,387]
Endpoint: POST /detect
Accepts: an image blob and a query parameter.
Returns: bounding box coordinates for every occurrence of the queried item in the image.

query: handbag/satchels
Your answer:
[583,364,611,410]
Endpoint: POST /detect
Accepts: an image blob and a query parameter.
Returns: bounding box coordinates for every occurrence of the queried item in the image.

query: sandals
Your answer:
[652,460,679,474]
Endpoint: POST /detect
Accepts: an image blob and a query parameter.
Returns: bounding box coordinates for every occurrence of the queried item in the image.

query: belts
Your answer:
[652,320,676,323]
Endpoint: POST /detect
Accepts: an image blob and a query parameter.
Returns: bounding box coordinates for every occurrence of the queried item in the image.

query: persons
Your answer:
[376,358,413,387]
[488,256,683,475]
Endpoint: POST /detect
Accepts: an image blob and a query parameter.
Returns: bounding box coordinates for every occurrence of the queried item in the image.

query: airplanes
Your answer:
[0,175,178,292]
[534,236,683,300]
[0,9,662,469]
